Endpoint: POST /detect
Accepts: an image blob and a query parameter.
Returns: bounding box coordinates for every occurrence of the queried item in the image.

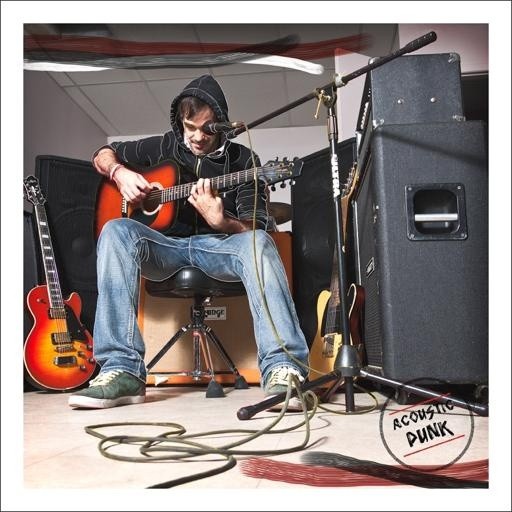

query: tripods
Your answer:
[236,89,489,423]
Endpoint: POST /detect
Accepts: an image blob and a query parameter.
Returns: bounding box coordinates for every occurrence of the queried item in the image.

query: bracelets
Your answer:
[109,163,123,180]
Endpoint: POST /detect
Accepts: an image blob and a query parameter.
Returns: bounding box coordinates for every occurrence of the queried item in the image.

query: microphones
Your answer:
[202,120,244,135]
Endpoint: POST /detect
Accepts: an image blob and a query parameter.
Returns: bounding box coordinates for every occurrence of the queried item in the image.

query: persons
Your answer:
[69,73,308,413]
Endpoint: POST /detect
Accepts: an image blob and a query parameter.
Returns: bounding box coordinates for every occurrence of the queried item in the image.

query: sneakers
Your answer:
[68,369,146,408]
[263,366,307,411]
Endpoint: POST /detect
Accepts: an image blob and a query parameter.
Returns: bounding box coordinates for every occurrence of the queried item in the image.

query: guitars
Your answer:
[94,156,303,242]
[308,161,366,389]
[23,173,96,390]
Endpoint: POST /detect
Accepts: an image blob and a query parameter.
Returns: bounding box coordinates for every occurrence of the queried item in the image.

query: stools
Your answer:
[144,266,247,399]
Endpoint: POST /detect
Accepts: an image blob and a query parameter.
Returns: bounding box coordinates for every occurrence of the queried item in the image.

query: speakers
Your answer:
[289,137,358,353]
[34,154,105,337]
[352,116,489,386]
[354,51,465,159]
